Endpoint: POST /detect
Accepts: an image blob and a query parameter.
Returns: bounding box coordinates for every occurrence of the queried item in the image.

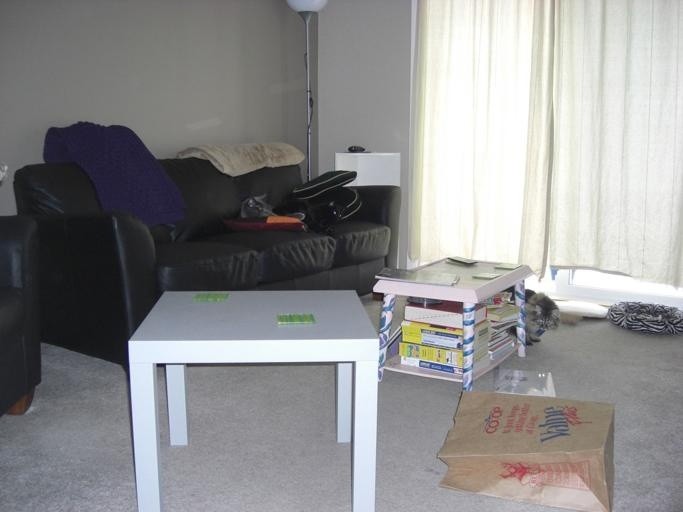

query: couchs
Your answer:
[0,213,49,421]
[12,155,393,375]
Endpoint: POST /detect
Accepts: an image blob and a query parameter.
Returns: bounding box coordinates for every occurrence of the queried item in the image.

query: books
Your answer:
[398,294,520,374]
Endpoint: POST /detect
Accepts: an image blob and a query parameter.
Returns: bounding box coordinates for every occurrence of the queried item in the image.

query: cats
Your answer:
[240,194,276,220]
[499,285,560,346]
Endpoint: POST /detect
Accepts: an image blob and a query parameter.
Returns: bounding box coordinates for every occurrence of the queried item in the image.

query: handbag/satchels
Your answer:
[293,169,362,233]
[435,389,616,512]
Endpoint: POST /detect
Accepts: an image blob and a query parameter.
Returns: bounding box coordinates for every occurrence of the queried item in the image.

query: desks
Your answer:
[127,290,383,512]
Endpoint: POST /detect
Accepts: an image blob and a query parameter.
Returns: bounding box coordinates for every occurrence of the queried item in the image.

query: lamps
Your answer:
[283,1,329,180]
